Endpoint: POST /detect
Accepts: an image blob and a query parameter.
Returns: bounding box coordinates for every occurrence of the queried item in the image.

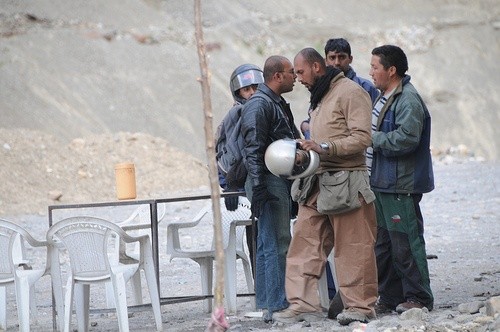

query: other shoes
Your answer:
[272,308,302,321]
[338,311,369,325]
[374,299,391,313]
[396,301,424,312]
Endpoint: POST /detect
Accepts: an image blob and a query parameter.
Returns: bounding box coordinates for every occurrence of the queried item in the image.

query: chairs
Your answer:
[0,196,338,332]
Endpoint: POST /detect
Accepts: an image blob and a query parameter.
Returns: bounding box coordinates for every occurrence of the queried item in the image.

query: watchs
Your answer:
[320,143,329,154]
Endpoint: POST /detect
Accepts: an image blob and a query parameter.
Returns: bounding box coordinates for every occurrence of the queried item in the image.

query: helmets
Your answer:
[265,138,321,180]
[230,63,263,104]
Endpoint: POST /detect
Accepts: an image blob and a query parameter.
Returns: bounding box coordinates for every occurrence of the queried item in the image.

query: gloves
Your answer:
[236,161,248,183]
[249,185,279,219]
[222,187,239,211]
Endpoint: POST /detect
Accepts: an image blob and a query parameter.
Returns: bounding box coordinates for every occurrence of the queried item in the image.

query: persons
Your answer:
[215,62,264,279]
[273,38,379,325]
[370,45,435,316]
[240,55,303,322]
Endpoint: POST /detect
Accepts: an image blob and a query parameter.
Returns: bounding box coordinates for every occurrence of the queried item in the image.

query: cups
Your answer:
[113,163,136,200]
